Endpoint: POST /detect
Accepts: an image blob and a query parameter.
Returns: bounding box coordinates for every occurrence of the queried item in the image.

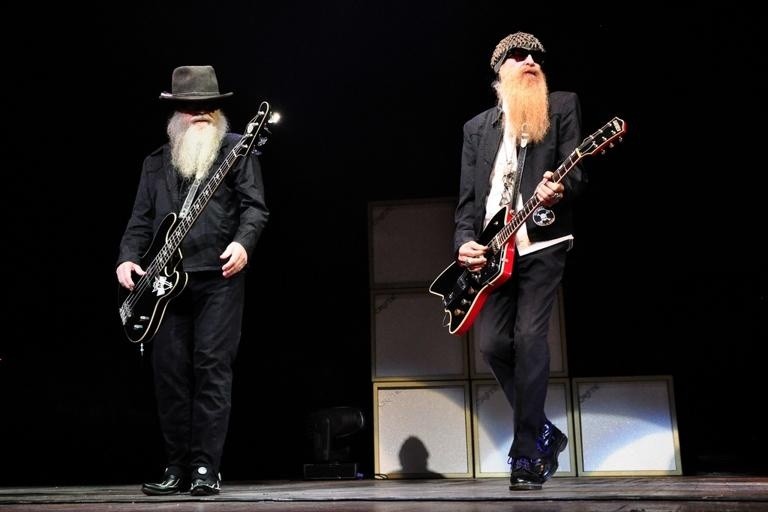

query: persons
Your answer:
[115,66,270,496]
[456,32,590,490]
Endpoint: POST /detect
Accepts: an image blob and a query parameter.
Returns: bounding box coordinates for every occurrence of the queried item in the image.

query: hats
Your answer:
[490,32,545,69]
[159,65,234,101]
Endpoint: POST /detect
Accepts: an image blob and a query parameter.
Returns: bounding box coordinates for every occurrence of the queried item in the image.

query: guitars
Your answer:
[116,99,272,344]
[429,116,626,336]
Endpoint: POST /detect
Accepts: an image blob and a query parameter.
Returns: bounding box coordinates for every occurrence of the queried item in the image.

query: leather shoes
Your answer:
[142,475,221,496]
[509,417,567,491]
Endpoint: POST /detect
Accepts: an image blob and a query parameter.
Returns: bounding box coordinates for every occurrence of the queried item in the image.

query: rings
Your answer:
[466,258,471,266]
[551,193,560,201]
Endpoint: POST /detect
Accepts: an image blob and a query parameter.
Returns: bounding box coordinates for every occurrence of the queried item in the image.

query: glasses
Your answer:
[507,48,544,66]
